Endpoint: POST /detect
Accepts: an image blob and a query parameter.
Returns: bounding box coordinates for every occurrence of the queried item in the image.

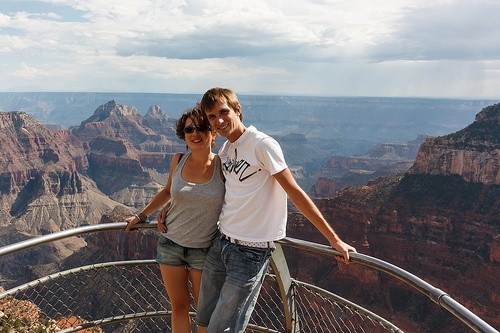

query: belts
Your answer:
[219,230,277,252]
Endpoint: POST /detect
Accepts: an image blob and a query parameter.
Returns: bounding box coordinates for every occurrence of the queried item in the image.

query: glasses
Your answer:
[182,125,213,134]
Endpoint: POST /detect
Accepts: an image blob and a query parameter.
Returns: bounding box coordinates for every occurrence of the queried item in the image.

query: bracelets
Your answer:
[133,212,147,223]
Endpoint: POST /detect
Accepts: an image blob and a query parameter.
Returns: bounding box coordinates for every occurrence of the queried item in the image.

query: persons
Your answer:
[194,87,357,333]
[125,107,227,333]
